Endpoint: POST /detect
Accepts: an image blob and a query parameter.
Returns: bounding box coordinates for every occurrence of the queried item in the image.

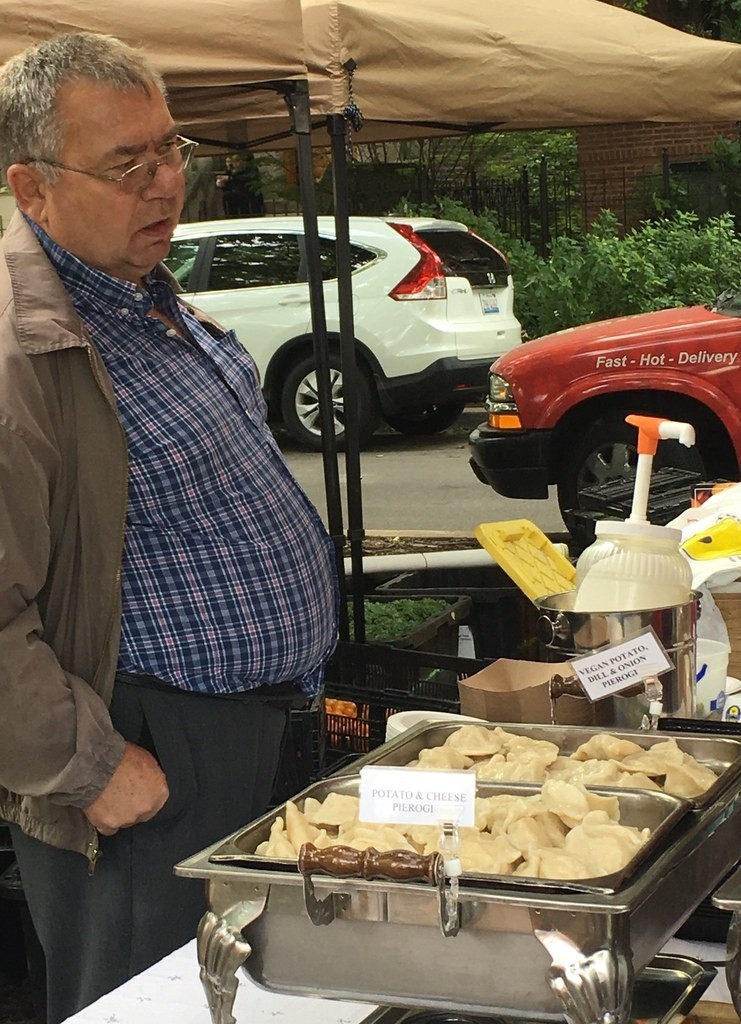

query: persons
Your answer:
[0,28,341,1024]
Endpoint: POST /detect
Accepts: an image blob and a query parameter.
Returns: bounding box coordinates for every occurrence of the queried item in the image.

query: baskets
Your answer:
[323,644,496,754]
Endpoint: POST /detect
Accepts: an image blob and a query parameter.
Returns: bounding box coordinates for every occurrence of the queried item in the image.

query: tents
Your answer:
[0,0,741,646]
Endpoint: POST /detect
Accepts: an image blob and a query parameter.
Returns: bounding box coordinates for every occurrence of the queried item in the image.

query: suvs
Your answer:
[167,216,524,455]
[466,290,741,549]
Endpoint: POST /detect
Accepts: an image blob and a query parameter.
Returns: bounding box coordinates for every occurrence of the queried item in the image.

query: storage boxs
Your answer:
[0,860,47,1024]
[345,593,471,685]
[345,558,580,664]
[560,467,734,549]
[305,642,496,785]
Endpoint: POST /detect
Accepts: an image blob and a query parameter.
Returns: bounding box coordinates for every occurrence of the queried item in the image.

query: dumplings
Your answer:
[252,725,718,881]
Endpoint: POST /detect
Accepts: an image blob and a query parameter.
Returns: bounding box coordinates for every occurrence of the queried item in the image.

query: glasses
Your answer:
[14,133,197,190]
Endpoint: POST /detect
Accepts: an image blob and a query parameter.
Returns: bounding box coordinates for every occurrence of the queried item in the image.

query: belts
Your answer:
[115,671,303,699]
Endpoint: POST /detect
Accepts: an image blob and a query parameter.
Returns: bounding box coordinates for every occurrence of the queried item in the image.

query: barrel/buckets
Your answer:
[696,637,728,722]
[533,589,703,720]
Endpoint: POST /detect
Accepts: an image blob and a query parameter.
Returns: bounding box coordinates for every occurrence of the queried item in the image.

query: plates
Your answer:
[726,676,741,695]
